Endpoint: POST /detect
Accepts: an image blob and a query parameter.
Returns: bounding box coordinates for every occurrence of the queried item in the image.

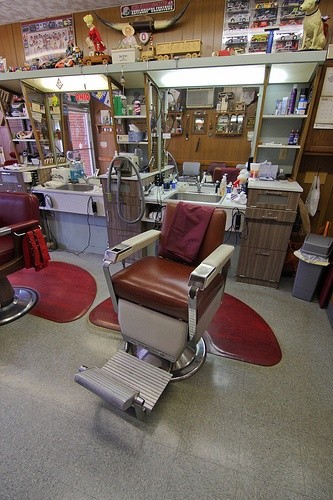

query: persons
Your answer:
[83,13,106,52]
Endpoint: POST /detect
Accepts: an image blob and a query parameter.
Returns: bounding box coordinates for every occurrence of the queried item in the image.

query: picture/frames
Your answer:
[191,114,207,135]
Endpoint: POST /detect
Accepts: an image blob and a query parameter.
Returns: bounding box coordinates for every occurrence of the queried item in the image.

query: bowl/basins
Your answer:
[128,131,145,142]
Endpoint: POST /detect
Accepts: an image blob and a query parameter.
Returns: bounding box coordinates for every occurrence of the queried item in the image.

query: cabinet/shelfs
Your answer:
[257,114,306,149]
[6,116,49,142]
[102,178,147,264]
[235,189,301,288]
[113,115,148,144]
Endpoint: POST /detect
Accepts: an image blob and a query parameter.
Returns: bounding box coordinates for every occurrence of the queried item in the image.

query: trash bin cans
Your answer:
[292,233,333,302]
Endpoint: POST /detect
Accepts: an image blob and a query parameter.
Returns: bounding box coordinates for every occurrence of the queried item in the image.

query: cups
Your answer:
[249,163,260,178]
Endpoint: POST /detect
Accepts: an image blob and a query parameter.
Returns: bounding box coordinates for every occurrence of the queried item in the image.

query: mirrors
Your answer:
[45,89,121,179]
[157,84,260,184]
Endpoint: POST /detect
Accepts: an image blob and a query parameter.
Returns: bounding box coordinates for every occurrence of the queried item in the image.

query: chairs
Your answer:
[74,201,283,418]
[0,191,96,323]
[213,168,242,184]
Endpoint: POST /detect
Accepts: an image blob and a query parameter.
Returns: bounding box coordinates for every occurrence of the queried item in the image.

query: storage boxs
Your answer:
[111,48,140,64]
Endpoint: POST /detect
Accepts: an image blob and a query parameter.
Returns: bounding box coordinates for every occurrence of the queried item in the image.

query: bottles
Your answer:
[275,83,307,145]
[70,158,84,182]
[134,100,140,115]
[238,172,248,193]
